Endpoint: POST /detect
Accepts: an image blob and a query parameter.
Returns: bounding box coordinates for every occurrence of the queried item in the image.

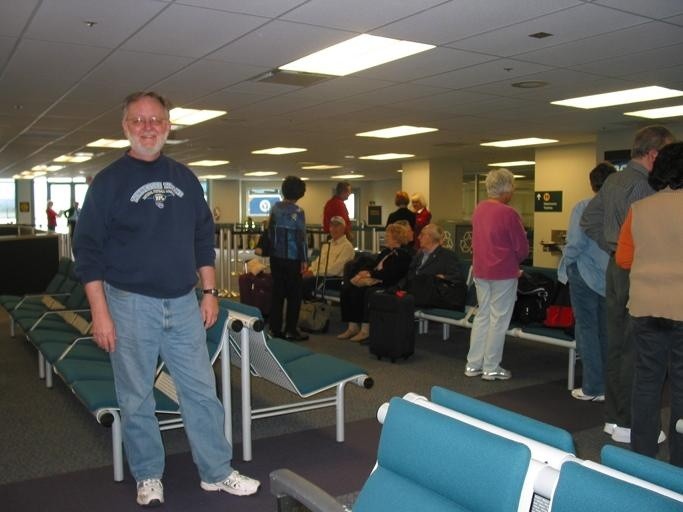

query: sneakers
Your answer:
[482,366,511,381]
[604,424,617,435]
[572,387,605,402]
[611,427,667,445]
[464,366,480,378]
[199,470,261,496]
[136,478,164,506]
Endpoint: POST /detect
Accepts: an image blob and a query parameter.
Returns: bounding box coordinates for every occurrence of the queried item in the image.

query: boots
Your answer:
[350,323,370,342]
[336,323,359,340]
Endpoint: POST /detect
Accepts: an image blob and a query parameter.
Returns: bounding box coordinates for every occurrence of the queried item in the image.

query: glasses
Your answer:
[125,117,169,126]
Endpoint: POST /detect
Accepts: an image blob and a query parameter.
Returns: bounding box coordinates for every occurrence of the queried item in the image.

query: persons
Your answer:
[465,169,530,380]
[579,125,674,445]
[364,223,467,328]
[391,214,415,246]
[300,215,356,302]
[382,190,416,232]
[557,162,617,402]
[342,224,412,342]
[320,178,356,247]
[63,201,81,237]
[251,176,312,343]
[44,200,60,234]
[408,194,431,235]
[615,142,682,465]
[69,93,263,506]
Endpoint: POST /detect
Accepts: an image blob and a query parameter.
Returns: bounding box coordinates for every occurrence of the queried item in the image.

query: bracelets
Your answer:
[202,287,220,296]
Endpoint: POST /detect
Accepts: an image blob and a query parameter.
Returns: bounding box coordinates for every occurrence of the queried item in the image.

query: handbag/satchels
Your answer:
[255,233,270,257]
[415,275,468,312]
[512,288,548,323]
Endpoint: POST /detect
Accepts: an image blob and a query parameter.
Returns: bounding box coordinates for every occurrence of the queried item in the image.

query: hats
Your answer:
[330,216,344,225]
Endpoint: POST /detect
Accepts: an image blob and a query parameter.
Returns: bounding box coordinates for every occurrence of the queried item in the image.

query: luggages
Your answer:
[239,258,255,305]
[251,270,275,326]
[368,284,415,364]
[299,302,331,332]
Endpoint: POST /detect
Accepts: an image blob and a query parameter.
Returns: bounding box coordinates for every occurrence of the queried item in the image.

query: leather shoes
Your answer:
[267,326,282,336]
[544,306,573,327]
[284,329,307,340]
